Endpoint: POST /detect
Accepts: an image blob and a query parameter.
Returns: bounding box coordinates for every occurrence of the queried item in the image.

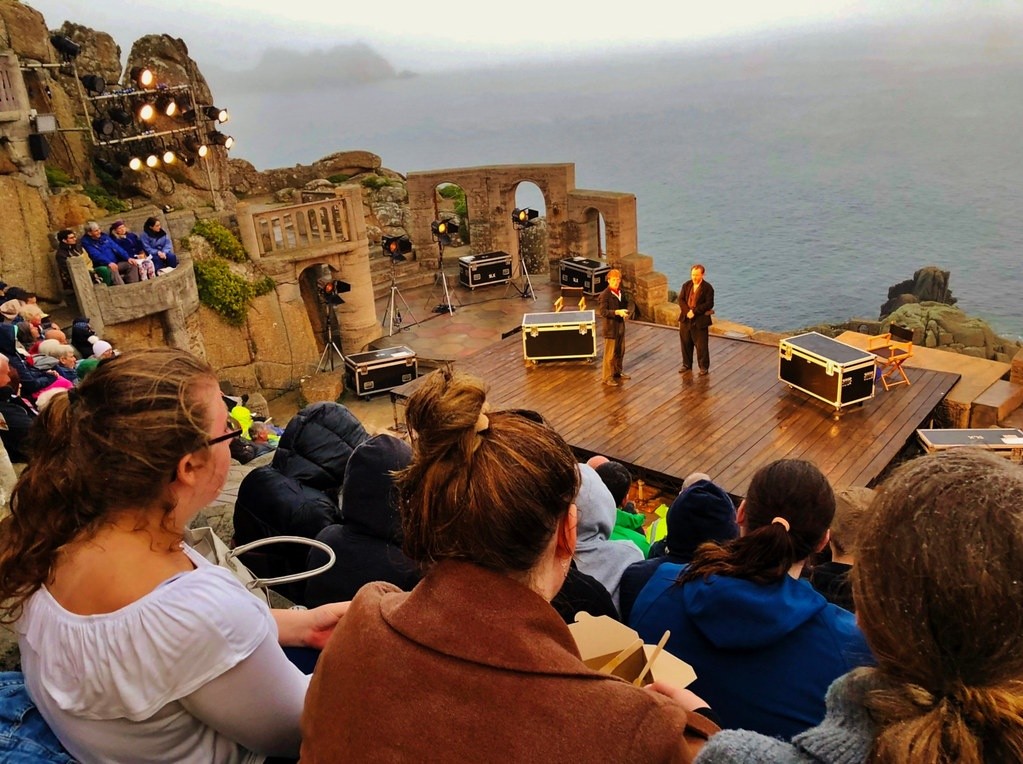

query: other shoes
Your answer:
[605,379,619,387]
[699,370,708,376]
[617,373,631,380]
[678,366,690,374]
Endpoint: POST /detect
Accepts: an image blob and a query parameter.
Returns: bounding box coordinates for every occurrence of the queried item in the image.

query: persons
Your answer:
[599,268,631,387]
[55,217,177,289]
[0,280,1023,763]
[677,264,715,375]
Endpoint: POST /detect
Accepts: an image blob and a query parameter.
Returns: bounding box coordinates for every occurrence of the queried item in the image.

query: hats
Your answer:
[87,335,113,359]
[17,304,43,321]
[112,220,125,230]
[827,485,878,566]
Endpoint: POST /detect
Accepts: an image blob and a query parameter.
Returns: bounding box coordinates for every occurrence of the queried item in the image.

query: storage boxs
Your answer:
[915,425,1023,461]
[558,253,611,295]
[459,249,514,289]
[344,345,417,397]
[776,329,877,417]
[521,310,598,365]
[569,611,698,689]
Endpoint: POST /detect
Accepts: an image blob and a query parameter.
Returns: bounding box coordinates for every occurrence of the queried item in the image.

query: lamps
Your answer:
[84,66,233,177]
[317,276,352,306]
[29,108,59,133]
[381,233,412,261]
[50,34,82,58]
[512,207,539,228]
[27,133,50,161]
[430,217,459,244]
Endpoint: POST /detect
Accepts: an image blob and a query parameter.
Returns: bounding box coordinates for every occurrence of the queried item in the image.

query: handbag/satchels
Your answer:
[179,525,337,609]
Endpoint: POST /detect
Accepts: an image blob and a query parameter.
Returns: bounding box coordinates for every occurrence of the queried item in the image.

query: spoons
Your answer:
[633,630,670,687]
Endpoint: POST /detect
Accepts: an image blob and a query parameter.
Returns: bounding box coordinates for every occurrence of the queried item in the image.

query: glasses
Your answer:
[170,413,243,485]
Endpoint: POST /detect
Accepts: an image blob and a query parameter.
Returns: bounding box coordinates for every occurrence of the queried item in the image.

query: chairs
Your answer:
[552,296,587,311]
[866,333,914,390]
